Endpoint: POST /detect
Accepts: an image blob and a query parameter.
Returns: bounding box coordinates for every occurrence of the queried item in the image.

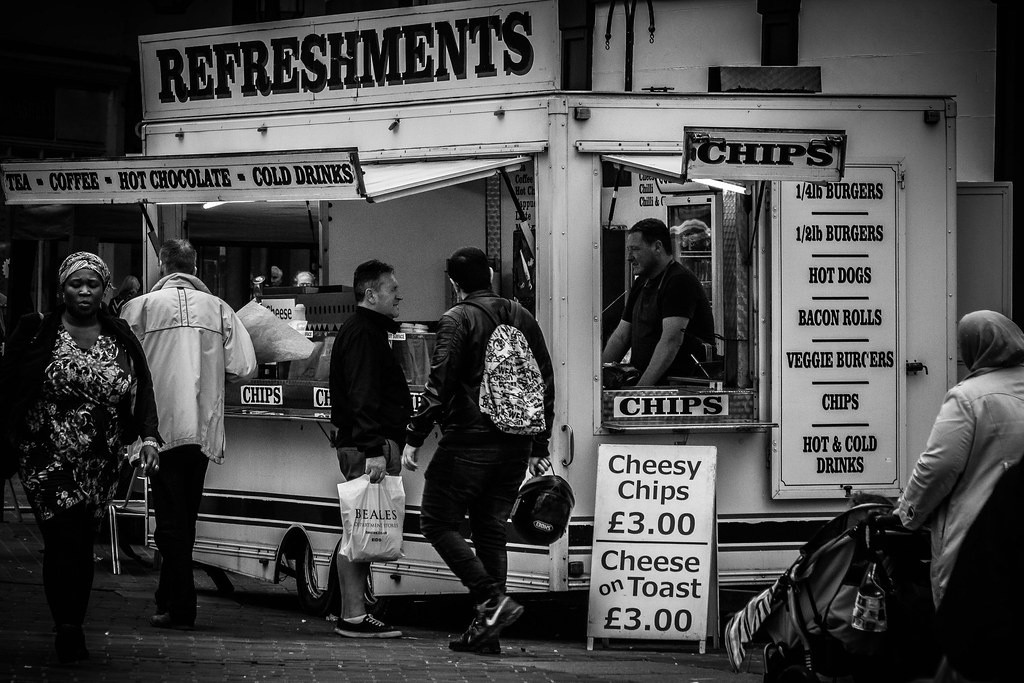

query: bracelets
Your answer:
[142,441,160,453]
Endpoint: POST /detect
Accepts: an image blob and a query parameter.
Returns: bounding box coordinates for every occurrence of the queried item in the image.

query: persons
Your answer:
[891,309,1024,613]
[119,238,257,630]
[602,217,718,387]
[329,259,414,639]
[402,246,555,656]
[0,250,165,664]
[108,275,142,319]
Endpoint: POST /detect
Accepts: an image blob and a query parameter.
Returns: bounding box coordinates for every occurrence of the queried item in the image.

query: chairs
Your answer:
[103,464,146,575]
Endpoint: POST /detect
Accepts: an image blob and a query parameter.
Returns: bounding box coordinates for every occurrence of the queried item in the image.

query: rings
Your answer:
[155,465,159,469]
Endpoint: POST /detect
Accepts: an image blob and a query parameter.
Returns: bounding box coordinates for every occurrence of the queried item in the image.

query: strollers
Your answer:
[758,503,948,683]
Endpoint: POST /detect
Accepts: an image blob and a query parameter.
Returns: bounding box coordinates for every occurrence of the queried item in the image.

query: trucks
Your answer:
[0,0,1015,623]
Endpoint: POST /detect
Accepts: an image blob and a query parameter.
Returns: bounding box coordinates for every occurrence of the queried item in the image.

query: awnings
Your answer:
[0,147,532,206]
[603,125,848,183]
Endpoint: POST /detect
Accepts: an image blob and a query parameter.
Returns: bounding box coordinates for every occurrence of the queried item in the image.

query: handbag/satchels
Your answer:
[336,474,403,561]
[851,563,888,632]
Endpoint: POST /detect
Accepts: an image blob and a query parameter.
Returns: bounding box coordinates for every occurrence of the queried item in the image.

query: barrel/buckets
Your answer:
[399,333,438,393]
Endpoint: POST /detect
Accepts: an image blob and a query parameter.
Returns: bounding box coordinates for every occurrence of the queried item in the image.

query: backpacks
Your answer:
[448,299,547,435]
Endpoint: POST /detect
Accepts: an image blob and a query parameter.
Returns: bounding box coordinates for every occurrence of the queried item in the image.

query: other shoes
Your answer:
[150,608,195,629]
[52,623,90,663]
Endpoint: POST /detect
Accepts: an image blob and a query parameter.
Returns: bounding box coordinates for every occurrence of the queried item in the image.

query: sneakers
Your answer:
[449,592,523,654]
[335,613,402,637]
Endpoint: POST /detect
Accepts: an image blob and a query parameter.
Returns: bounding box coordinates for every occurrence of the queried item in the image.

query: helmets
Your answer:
[510,475,575,546]
